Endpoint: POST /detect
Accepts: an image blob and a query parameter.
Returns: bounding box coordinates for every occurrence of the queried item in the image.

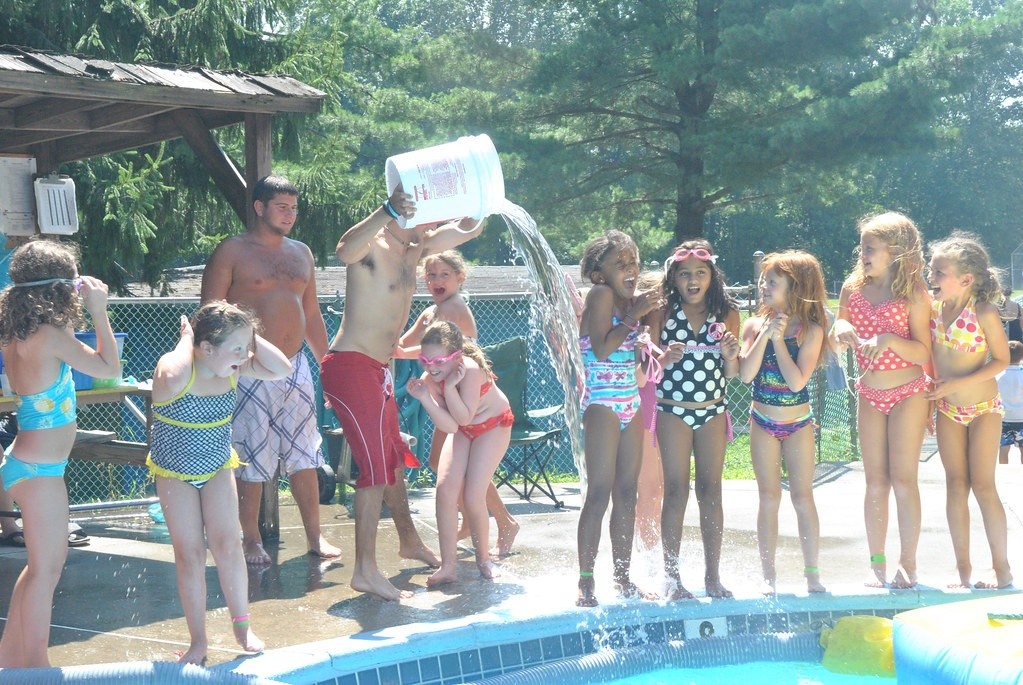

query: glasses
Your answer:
[419,349,463,366]
[672,248,712,261]
[73,278,83,289]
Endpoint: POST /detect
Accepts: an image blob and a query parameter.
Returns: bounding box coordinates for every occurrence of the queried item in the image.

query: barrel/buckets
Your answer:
[385,134,506,231]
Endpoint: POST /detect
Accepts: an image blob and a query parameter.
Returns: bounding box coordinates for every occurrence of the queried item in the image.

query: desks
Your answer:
[0,383,153,467]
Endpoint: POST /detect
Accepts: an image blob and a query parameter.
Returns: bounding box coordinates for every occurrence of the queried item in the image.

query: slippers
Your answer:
[0,530,26,547]
[67,523,91,546]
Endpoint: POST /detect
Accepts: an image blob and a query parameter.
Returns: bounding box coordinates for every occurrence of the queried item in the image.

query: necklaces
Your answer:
[383,224,405,244]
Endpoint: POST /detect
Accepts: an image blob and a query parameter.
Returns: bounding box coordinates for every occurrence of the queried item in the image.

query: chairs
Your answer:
[479,336,566,510]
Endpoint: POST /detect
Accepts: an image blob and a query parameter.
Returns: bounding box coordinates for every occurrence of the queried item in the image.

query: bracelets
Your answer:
[626,314,637,322]
[382,198,401,219]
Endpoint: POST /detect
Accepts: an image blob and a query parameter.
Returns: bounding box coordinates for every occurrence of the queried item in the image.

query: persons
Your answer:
[922,229,1014,590]
[0,444,26,546]
[392,249,520,587]
[739,250,831,595]
[986,265,1023,465]
[0,237,122,669]
[148,302,296,663]
[575,229,664,607]
[827,211,932,588]
[200,176,344,565]
[641,237,742,600]
[318,184,489,603]
